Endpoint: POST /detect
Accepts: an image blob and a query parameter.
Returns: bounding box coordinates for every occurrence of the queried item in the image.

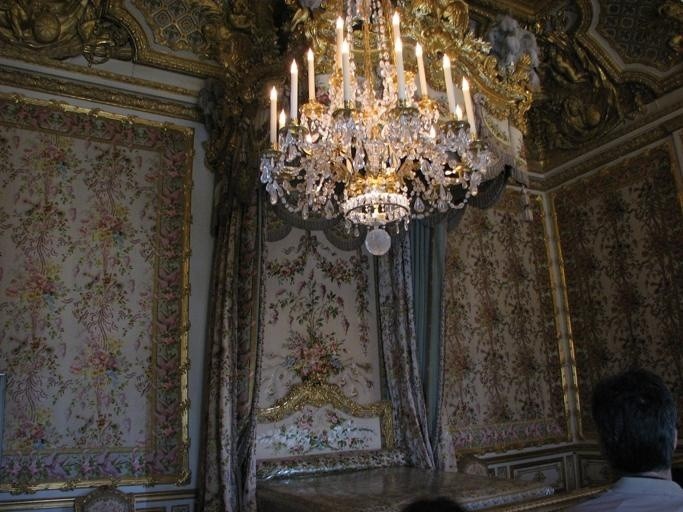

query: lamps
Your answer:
[259,2,495,255]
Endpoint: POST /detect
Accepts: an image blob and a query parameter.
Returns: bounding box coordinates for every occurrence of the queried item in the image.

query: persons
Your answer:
[565,368,683,511]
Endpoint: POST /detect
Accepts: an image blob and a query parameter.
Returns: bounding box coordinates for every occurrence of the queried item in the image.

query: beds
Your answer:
[255,369,556,511]
